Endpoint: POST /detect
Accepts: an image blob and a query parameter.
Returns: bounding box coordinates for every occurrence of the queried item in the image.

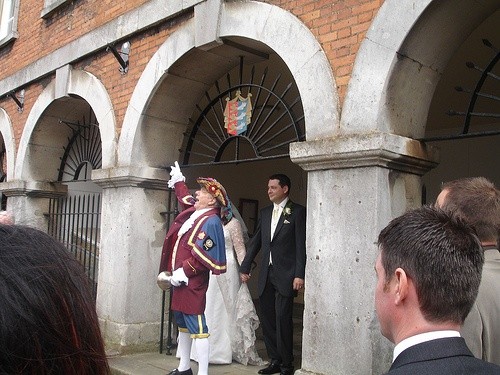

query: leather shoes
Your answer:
[165,367,193,375]
[280,360,293,375]
[258,359,282,375]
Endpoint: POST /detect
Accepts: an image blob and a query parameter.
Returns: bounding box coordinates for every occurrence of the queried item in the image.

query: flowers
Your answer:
[279,202,294,219]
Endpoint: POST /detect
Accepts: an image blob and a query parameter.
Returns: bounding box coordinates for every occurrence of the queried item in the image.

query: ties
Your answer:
[268,204,281,266]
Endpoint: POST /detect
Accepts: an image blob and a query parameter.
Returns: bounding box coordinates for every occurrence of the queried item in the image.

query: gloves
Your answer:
[170,267,189,288]
[167,159,186,189]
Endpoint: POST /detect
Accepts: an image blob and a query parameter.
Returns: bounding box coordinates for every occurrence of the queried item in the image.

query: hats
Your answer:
[196,174,228,207]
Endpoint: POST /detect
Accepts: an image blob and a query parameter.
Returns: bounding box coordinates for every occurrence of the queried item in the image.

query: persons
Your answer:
[175,201,251,366]
[0,223,113,375]
[159,161,228,375]
[238,174,307,375]
[374,206,500,375]
[434,176,500,366]
